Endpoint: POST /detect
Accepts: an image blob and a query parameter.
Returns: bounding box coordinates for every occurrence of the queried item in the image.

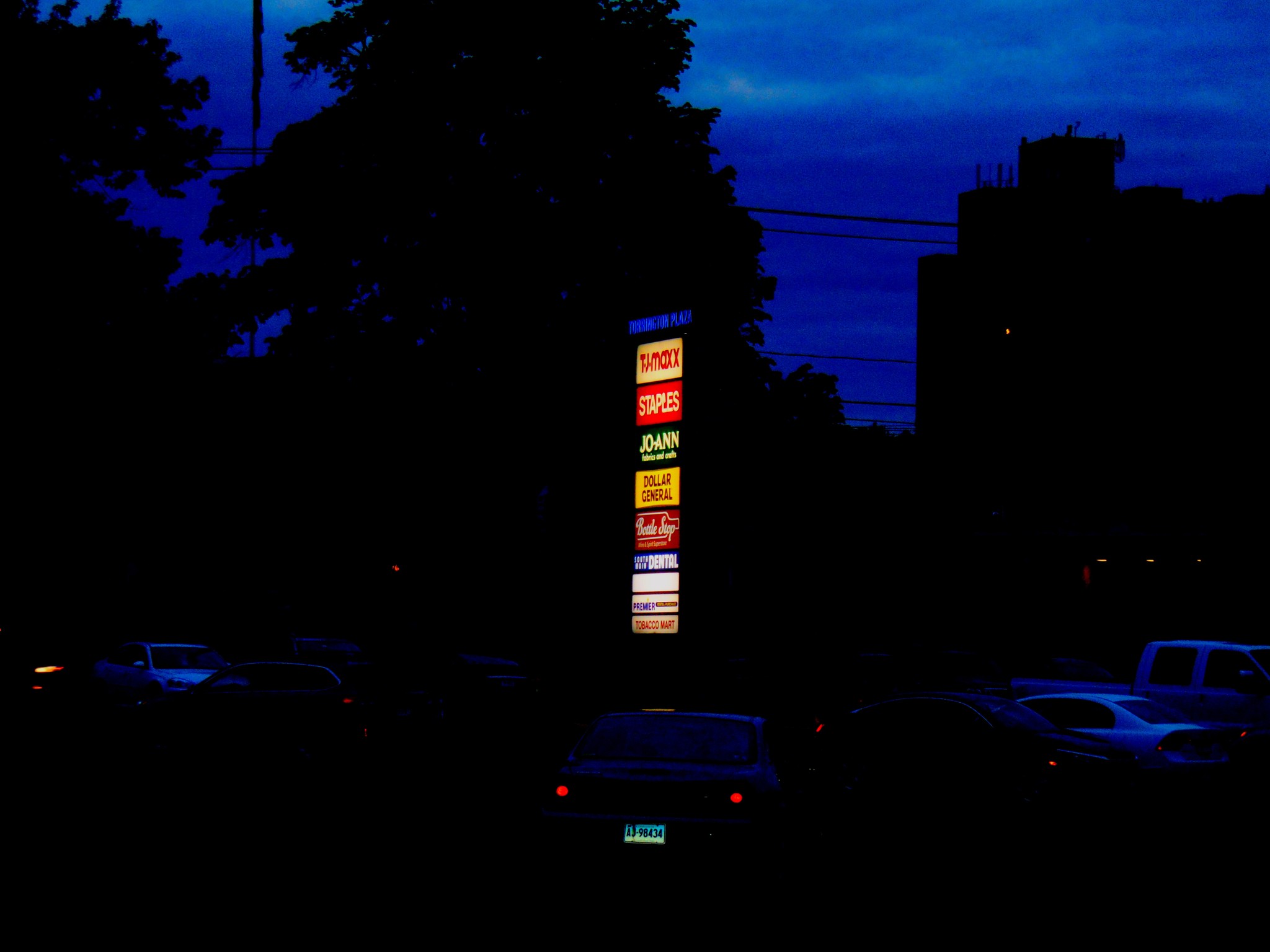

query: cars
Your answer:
[818,639,1270,845]
[22,635,541,757]
[536,707,810,862]
